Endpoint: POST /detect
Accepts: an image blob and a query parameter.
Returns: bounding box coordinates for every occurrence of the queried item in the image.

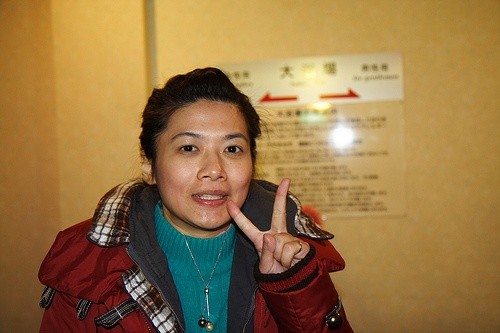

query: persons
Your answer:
[39,67,353,333]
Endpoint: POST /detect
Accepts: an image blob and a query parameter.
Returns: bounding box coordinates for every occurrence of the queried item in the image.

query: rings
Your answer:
[296,239,303,254]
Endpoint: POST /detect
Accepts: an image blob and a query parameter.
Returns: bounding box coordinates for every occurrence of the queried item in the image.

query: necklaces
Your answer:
[178,228,229,331]
